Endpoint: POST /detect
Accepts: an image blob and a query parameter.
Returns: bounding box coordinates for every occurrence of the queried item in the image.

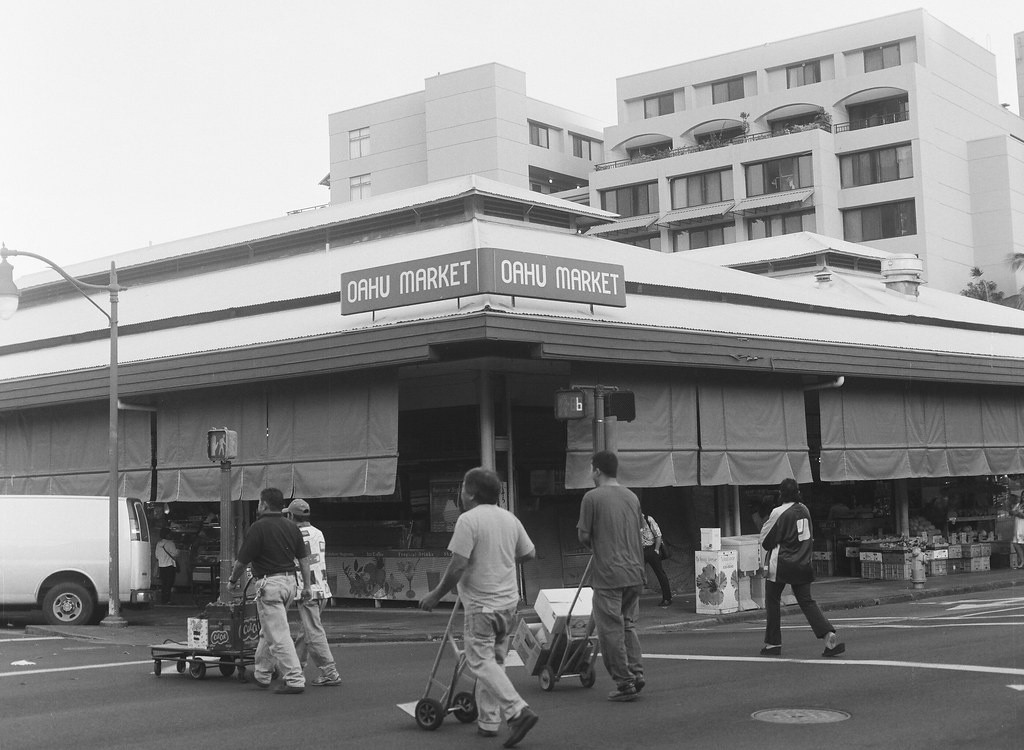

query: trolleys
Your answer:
[149,576,281,684]
[538,551,602,692]
[414,594,478,732]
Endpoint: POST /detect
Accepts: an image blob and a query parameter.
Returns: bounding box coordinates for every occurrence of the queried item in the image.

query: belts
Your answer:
[255,572,295,581]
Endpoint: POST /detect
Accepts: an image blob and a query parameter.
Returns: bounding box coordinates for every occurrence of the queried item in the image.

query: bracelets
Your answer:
[229,576,236,584]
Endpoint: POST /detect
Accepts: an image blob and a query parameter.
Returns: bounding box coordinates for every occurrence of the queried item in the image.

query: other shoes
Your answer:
[503,707,539,747]
[608,684,639,701]
[244,670,269,688]
[311,673,342,685]
[272,686,305,694]
[161,601,176,605]
[657,599,672,606]
[477,725,498,736]
[633,671,646,693]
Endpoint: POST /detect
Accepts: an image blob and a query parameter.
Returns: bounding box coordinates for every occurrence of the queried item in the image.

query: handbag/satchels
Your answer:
[659,542,671,560]
[175,561,180,573]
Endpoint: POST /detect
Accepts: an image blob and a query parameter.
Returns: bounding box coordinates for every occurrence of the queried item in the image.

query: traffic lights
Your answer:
[206,429,239,463]
[554,389,588,422]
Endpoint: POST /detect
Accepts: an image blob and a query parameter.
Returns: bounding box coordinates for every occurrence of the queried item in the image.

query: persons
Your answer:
[576,450,647,701]
[418,467,538,750]
[155,528,177,605]
[759,479,846,656]
[639,512,672,607]
[282,498,342,687]
[227,487,312,694]
[1008,490,1024,569]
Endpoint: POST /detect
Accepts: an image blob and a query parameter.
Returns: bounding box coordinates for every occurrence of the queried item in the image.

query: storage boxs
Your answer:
[511,587,594,675]
[206,603,261,651]
[812,537,991,580]
[187,617,208,650]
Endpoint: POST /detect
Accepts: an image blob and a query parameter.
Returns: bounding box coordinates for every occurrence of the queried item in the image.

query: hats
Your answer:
[281,499,310,515]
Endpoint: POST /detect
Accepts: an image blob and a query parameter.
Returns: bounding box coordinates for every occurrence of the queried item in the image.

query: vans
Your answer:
[1,493,157,629]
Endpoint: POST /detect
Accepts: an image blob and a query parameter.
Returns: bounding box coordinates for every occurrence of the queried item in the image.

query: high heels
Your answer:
[760,645,782,655]
[821,643,845,657]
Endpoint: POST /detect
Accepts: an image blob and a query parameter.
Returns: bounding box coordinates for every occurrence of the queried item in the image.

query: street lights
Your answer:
[1,239,131,629]
